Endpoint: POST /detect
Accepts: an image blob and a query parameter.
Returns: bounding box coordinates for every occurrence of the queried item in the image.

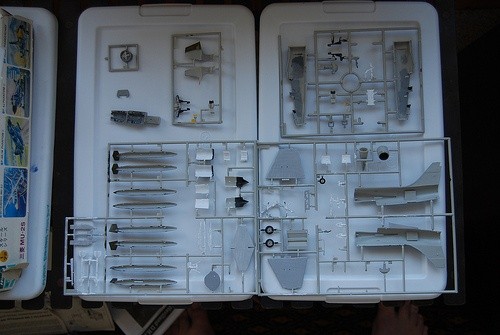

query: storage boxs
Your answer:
[70,1,451,309]
[0,5,59,305]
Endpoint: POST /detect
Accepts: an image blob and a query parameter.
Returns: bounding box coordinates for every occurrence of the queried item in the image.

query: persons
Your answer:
[154,303,428,335]
